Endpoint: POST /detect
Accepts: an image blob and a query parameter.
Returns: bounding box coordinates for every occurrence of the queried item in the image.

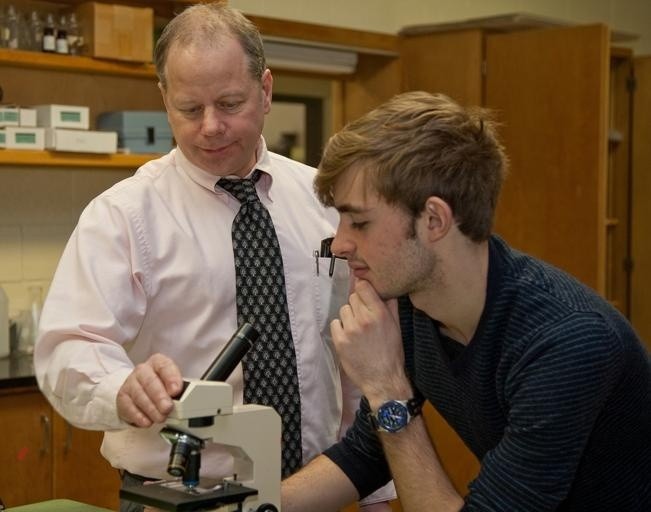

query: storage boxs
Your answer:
[74,2,155,63]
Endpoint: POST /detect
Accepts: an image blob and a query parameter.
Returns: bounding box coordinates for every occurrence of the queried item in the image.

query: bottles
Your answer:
[16,286,43,357]
[0,0,85,56]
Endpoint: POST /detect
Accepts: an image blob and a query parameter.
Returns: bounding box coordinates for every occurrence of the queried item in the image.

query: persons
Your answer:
[32,1,403,511]
[281,88,648,508]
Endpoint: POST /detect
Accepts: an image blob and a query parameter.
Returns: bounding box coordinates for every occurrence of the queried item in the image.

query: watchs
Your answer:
[366,393,424,437]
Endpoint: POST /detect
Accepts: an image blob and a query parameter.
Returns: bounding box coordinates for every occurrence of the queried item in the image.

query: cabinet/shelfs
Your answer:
[0,46,178,171]
[386,21,651,512]
[1,386,123,509]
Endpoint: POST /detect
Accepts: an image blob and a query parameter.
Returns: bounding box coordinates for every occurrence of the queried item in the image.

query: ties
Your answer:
[216,169,304,483]
